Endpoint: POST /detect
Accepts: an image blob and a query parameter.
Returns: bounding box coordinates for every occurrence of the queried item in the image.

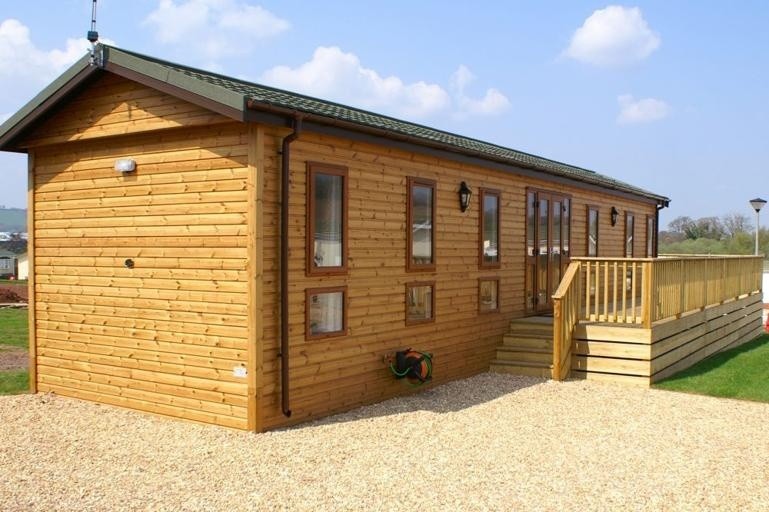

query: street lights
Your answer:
[748,198,767,254]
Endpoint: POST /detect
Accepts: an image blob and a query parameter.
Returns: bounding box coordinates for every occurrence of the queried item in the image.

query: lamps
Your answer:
[611,207,619,225]
[459,182,472,212]
